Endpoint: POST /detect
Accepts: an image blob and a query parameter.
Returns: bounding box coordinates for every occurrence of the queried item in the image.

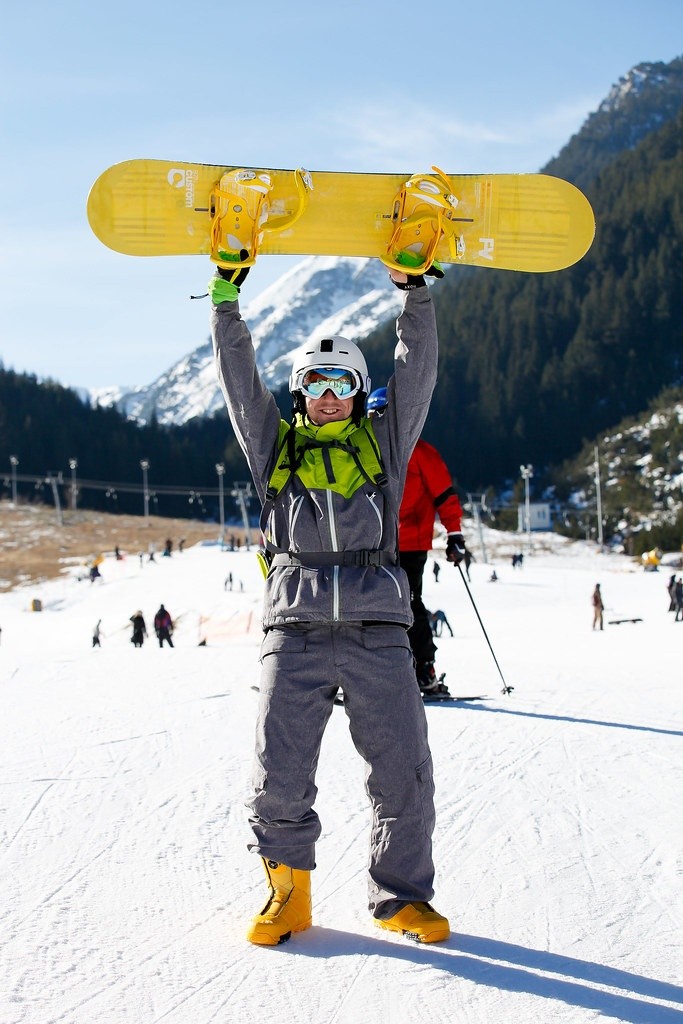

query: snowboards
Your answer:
[85,156,597,273]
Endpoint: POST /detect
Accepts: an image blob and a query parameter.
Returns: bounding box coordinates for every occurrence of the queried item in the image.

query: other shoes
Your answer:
[418,663,439,690]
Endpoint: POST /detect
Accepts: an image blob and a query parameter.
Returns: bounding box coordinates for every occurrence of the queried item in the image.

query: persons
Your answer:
[130,610,150,648]
[92,619,102,647]
[491,571,497,579]
[363,383,471,701]
[667,575,683,621]
[512,552,523,566]
[114,545,120,557]
[179,538,185,552]
[154,604,175,647]
[229,534,249,551]
[433,561,440,582]
[591,584,605,631]
[148,539,156,560]
[208,247,452,945]
[166,538,173,553]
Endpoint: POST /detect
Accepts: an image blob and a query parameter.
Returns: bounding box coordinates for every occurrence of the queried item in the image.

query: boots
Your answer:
[247,855,311,944]
[374,896,448,945]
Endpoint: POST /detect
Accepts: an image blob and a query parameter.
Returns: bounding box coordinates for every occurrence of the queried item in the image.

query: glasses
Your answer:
[297,365,364,400]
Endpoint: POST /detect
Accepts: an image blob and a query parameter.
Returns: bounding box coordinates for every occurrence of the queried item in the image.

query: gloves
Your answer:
[215,251,249,287]
[445,534,466,566]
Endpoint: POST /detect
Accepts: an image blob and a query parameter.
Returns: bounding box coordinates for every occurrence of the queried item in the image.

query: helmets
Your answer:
[288,335,371,397]
